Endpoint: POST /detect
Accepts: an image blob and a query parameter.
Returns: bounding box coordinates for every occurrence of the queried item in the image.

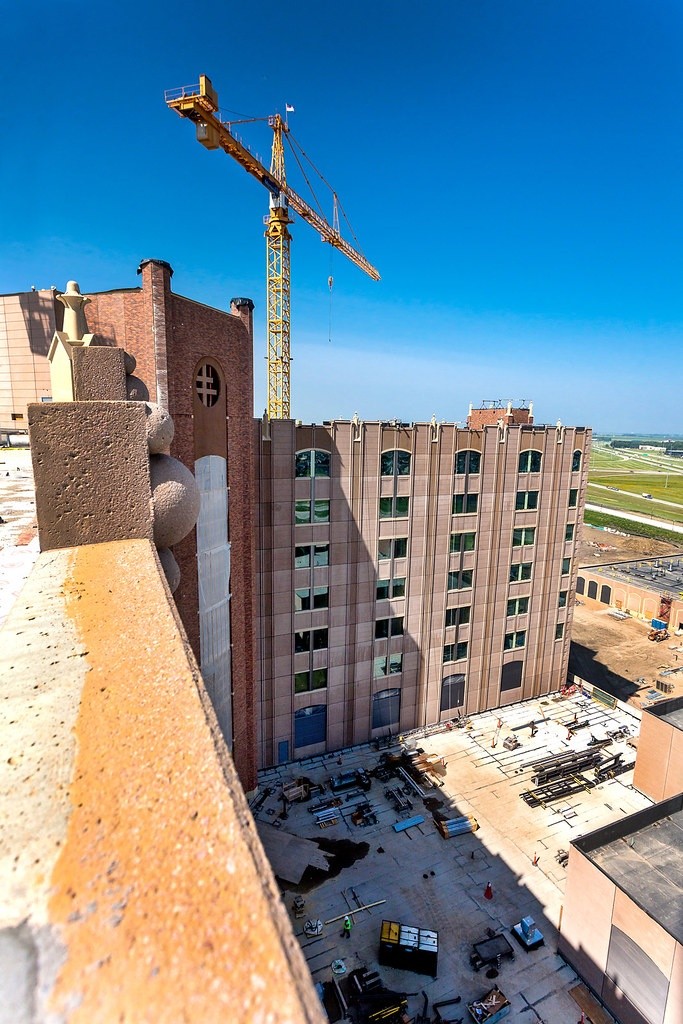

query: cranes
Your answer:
[162,75,382,417]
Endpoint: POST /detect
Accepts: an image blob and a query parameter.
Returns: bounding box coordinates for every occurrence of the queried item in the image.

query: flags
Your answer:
[286,103,295,113]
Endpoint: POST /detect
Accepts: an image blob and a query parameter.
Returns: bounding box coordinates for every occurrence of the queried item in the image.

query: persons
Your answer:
[340,916,351,939]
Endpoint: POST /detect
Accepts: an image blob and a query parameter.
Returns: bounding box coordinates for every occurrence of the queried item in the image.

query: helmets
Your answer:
[344,916,348,920]
